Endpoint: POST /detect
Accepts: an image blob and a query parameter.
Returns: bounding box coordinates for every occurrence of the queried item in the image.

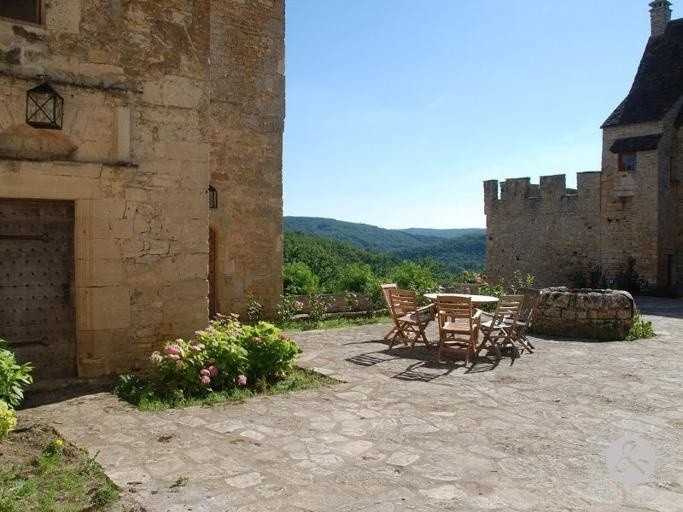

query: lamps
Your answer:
[208,186,218,209]
[25,73,64,130]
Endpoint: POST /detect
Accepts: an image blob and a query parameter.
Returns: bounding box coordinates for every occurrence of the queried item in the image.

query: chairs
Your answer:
[382,282,540,365]
